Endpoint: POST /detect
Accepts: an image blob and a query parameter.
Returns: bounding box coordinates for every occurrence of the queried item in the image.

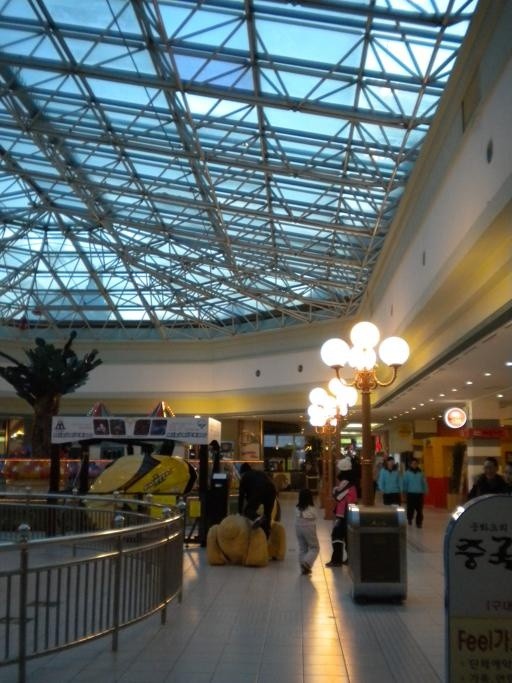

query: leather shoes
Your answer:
[325,560,342,566]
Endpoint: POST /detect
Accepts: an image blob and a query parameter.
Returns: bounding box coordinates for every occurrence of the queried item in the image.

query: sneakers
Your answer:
[301,562,312,572]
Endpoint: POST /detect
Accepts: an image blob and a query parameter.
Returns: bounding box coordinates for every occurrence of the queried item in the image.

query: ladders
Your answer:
[304,461,322,495]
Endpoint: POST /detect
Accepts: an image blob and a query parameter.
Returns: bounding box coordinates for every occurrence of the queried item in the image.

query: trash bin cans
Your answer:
[344,505,408,605]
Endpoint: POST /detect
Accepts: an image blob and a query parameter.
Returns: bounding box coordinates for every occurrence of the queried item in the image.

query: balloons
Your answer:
[0,459,100,485]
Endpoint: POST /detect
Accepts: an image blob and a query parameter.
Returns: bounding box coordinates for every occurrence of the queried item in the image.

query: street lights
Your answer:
[321,321,410,505]
[307,377,358,520]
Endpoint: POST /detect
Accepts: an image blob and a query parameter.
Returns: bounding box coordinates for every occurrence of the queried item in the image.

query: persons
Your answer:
[336,456,362,505]
[325,472,359,567]
[504,461,512,495]
[465,456,504,498]
[376,457,403,505]
[403,457,429,529]
[237,462,277,538]
[294,488,321,576]
